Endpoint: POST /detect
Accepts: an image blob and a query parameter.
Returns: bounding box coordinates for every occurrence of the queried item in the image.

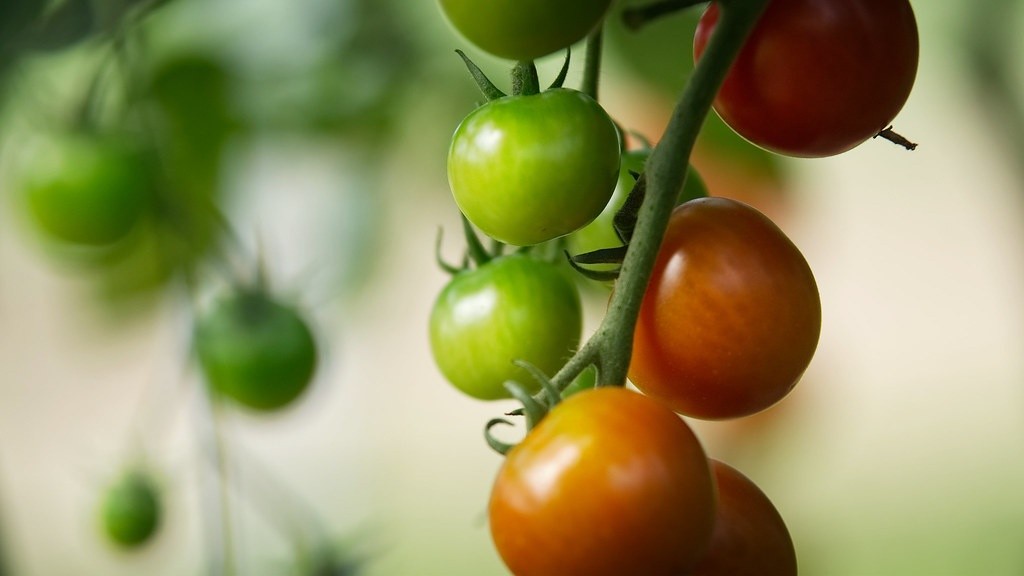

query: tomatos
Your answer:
[26,0,920,576]
